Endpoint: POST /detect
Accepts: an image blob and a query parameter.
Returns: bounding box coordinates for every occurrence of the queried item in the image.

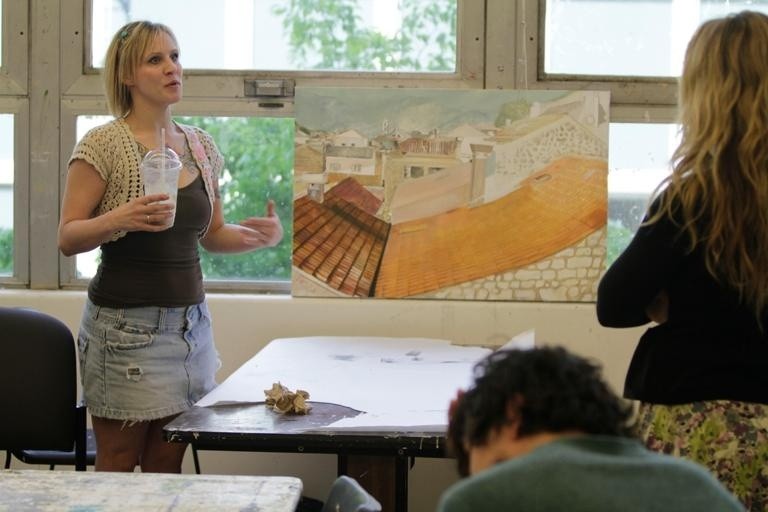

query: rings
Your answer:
[147,215,151,224]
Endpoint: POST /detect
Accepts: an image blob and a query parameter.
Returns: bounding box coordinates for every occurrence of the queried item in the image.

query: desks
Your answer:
[0,469,303,512]
[164,336,503,512]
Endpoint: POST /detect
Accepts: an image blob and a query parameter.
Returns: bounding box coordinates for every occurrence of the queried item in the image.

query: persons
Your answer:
[596,8,767,509]
[437,348,748,510]
[57,19,283,474]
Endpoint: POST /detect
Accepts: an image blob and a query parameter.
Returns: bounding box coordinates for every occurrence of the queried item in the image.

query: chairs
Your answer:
[0,306,205,475]
[321,476,382,512]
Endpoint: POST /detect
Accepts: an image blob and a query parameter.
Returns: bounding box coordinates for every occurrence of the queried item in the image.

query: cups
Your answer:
[140,145,183,230]
